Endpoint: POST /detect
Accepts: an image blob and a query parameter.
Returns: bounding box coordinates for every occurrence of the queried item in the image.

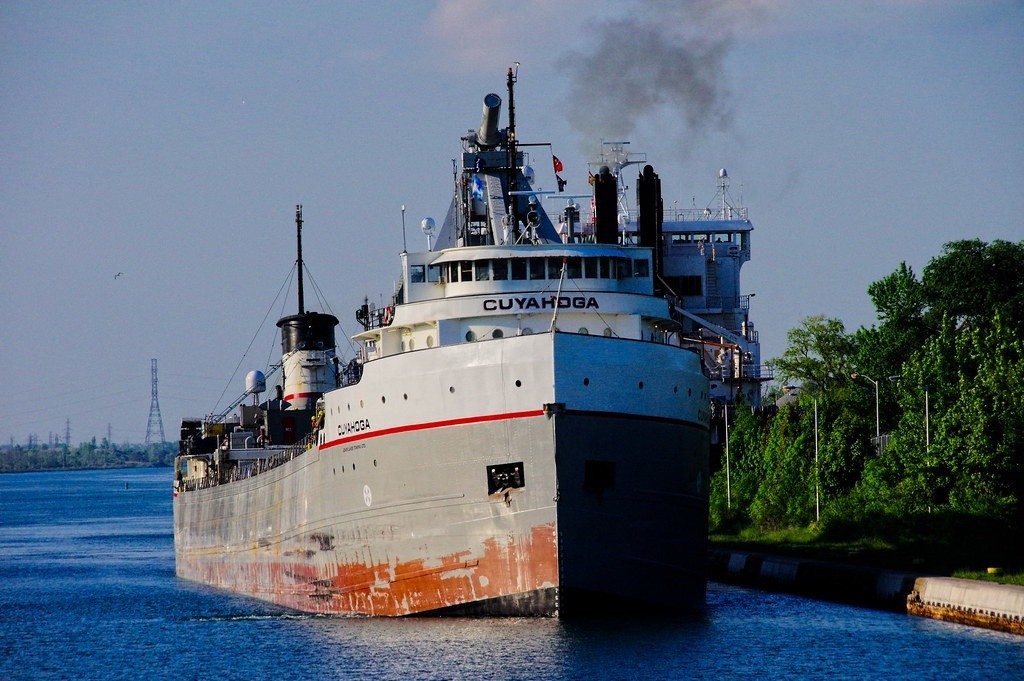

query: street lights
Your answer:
[783,386,819,522]
[889,376,930,513]
[851,373,879,453]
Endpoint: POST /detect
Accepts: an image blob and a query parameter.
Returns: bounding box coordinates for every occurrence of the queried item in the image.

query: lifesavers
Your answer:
[384,306,392,324]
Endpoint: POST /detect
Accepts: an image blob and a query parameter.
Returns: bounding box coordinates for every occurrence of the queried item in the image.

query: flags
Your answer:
[553,154,567,193]
[587,171,596,186]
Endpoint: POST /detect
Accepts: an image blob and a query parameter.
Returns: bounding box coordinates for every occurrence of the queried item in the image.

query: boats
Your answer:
[173,61,774,622]
